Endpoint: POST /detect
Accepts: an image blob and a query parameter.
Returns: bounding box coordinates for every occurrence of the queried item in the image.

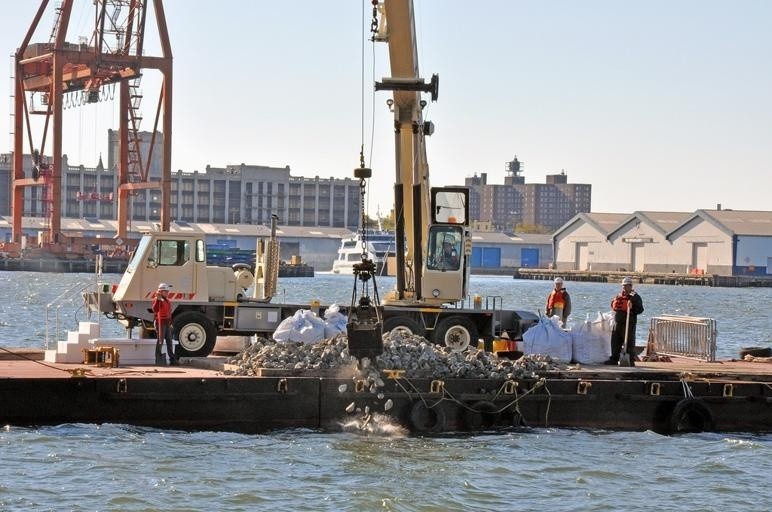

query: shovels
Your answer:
[619,300,630,367]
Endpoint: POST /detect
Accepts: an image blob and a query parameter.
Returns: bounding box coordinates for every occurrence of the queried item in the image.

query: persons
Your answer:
[545,276,571,328]
[153,284,181,365]
[436,239,457,270]
[603,277,644,367]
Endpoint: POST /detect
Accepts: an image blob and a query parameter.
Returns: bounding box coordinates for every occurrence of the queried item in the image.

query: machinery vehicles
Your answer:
[79,1,543,371]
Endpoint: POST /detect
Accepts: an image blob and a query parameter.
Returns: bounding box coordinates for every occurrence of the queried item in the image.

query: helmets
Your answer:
[621,278,632,285]
[555,278,563,284]
[158,284,169,291]
[443,238,452,245]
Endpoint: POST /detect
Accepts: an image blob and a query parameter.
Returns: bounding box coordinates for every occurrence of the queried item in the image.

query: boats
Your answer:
[332,203,456,273]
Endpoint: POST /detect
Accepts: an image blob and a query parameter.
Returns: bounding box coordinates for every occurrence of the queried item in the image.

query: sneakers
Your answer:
[603,359,618,365]
[630,361,635,366]
